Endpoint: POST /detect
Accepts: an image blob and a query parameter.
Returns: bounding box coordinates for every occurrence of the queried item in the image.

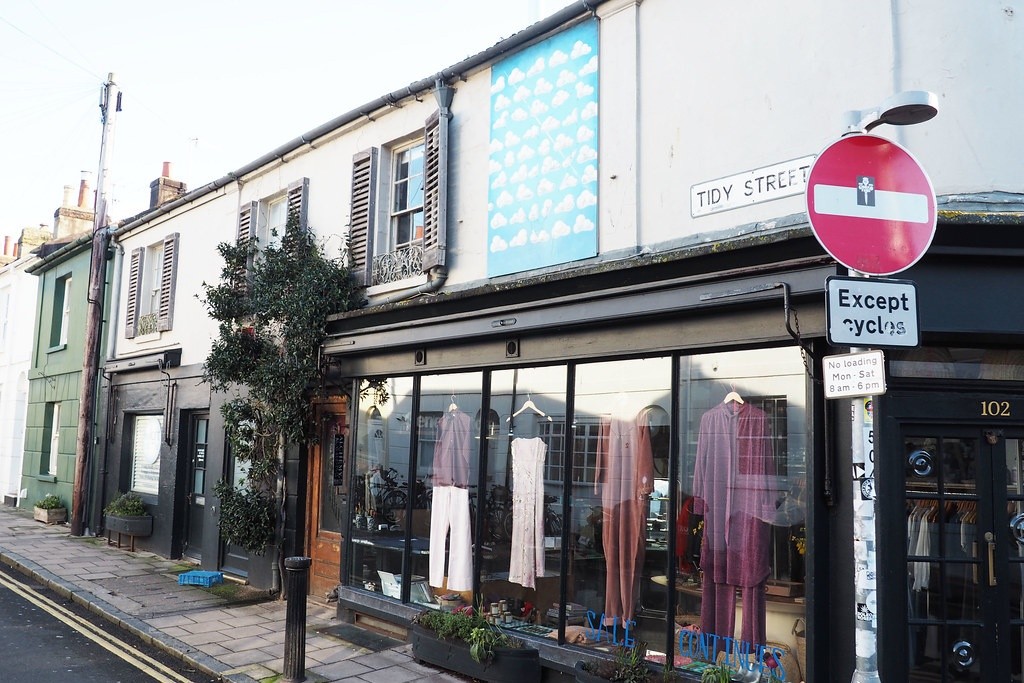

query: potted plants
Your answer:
[409,612,540,683]
[34,494,67,524]
[102,492,153,537]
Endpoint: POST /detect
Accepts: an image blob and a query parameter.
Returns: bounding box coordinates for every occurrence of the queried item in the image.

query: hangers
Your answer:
[912,497,1014,523]
[720,383,746,408]
[505,392,555,422]
[448,396,459,413]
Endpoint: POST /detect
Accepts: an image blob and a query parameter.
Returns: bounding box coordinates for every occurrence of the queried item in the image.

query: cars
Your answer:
[646,479,681,550]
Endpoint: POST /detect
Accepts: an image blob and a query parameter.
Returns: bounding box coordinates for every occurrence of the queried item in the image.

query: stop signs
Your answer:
[805,133,938,275]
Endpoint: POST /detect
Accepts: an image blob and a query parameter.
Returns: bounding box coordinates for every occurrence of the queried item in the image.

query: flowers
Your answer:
[792,529,806,557]
[590,641,677,683]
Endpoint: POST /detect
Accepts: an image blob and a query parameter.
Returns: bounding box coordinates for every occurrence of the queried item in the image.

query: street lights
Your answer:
[845,86,940,683]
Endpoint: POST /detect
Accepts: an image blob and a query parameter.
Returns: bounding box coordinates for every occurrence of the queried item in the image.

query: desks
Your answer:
[351,529,403,583]
[351,537,450,599]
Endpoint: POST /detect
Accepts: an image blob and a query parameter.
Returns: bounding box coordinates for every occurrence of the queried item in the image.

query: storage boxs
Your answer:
[395,574,424,601]
[434,570,573,619]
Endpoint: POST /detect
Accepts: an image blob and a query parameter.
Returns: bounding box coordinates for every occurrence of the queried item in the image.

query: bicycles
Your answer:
[351,467,562,544]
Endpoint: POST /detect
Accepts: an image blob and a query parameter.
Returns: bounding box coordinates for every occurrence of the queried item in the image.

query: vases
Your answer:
[575,661,623,683]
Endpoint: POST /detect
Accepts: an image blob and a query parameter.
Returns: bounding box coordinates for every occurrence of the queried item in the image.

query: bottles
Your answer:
[486,600,512,625]
